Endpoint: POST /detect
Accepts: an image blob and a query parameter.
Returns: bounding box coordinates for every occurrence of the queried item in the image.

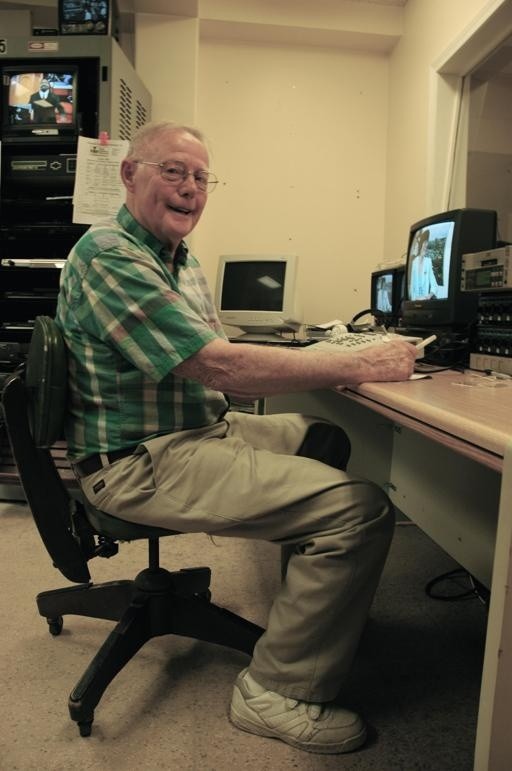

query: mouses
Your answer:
[292,339,299,344]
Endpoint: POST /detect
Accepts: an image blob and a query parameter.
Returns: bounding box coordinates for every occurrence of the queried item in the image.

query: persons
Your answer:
[30,79,65,124]
[409,230,438,302]
[377,278,392,313]
[51,121,417,755]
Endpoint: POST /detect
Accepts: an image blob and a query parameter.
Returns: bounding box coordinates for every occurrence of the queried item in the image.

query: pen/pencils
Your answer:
[415,334,438,350]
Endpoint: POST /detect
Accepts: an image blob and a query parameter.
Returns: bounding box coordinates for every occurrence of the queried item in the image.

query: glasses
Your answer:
[135,158,219,193]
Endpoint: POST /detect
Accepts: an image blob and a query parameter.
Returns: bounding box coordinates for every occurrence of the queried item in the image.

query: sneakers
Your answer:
[230,666,368,753]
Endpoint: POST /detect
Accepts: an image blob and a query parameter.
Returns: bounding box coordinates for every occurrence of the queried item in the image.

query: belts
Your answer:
[72,445,139,479]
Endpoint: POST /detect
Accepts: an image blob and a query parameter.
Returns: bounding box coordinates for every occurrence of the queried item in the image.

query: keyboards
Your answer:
[227,339,318,347]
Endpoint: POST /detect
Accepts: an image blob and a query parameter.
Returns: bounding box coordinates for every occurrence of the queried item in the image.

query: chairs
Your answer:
[0,314,266,737]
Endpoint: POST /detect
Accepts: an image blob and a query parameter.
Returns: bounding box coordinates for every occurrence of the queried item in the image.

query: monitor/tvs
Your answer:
[213,253,304,342]
[371,265,406,327]
[0,64,83,138]
[57,0,119,44]
[401,207,497,332]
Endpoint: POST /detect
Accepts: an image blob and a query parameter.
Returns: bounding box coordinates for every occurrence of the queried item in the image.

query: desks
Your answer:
[265,342,512,768]
